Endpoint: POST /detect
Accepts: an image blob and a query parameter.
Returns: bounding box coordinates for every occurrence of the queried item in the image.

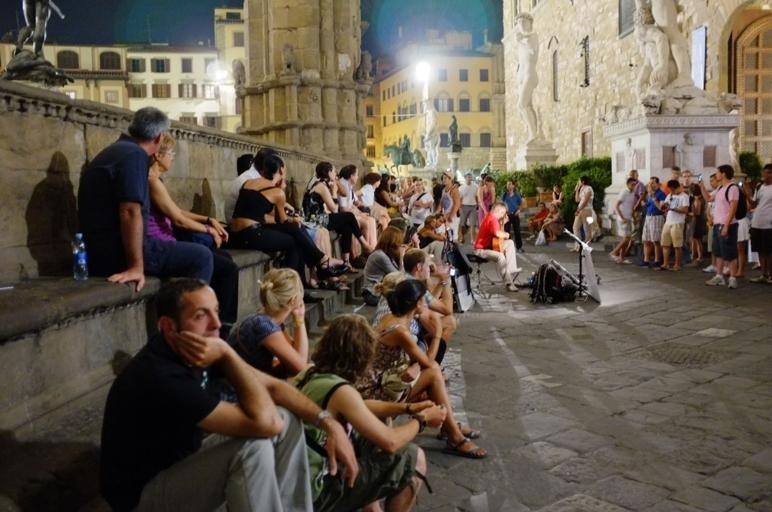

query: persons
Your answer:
[78,106,215,293]
[352,279,489,459]
[290,312,448,512]
[525,184,565,243]
[101,277,314,512]
[225,147,524,383]
[609,164,772,288]
[145,131,240,323]
[569,177,594,254]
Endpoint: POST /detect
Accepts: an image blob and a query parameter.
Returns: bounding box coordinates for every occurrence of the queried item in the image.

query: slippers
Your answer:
[727,271,745,278]
[610,252,620,259]
[616,259,632,264]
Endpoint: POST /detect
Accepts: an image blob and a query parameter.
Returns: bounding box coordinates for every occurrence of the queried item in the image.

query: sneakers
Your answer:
[516,233,558,253]
[749,263,772,285]
[459,239,465,243]
[661,255,704,271]
[471,240,475,244]
[651,261,661,271]
[633,261,650,268]
[704,264,738,288]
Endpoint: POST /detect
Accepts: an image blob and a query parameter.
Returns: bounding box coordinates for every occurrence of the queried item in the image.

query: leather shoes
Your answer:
[510,267,523,274]
[507,284,520,292]
[304,291,325,305]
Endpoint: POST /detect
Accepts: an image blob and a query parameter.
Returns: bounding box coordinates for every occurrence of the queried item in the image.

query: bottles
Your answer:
[530,272,536,285]
[71,232,89,281]
[299,208,305,221]
[310,214,316,224]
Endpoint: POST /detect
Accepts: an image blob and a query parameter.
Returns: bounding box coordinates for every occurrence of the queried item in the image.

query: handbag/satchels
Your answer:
[316,433,382,512]
[286,207,305,223]
[381,361,421,401]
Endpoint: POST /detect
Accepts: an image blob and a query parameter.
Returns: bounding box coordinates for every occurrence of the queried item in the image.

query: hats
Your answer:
[445,171,455,181]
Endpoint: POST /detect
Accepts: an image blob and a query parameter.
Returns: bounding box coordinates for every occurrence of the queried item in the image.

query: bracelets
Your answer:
[315,409,333,428]
[207,216,209,224]
[205,223,211,235]
[412,414,427,433]
[407,405,411,414]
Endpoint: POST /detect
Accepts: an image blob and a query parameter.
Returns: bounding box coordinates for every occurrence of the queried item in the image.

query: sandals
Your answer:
[447,437,489,459]
[440,421,482,441]
[309,248,374,291]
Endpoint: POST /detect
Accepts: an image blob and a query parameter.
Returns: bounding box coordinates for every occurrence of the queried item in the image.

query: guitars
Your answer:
[492,215,504,253]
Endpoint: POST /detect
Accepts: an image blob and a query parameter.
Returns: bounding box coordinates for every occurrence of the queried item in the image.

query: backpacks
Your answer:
[719,184,749,221]
[452,241,474,276]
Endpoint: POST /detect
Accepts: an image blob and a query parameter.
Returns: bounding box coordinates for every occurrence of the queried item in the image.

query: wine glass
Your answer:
[318,213,329,229]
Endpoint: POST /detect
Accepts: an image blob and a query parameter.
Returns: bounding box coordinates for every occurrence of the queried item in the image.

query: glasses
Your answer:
[159,152,176,160]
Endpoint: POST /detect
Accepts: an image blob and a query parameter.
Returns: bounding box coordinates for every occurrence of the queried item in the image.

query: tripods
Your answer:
[574,254,600,304]
